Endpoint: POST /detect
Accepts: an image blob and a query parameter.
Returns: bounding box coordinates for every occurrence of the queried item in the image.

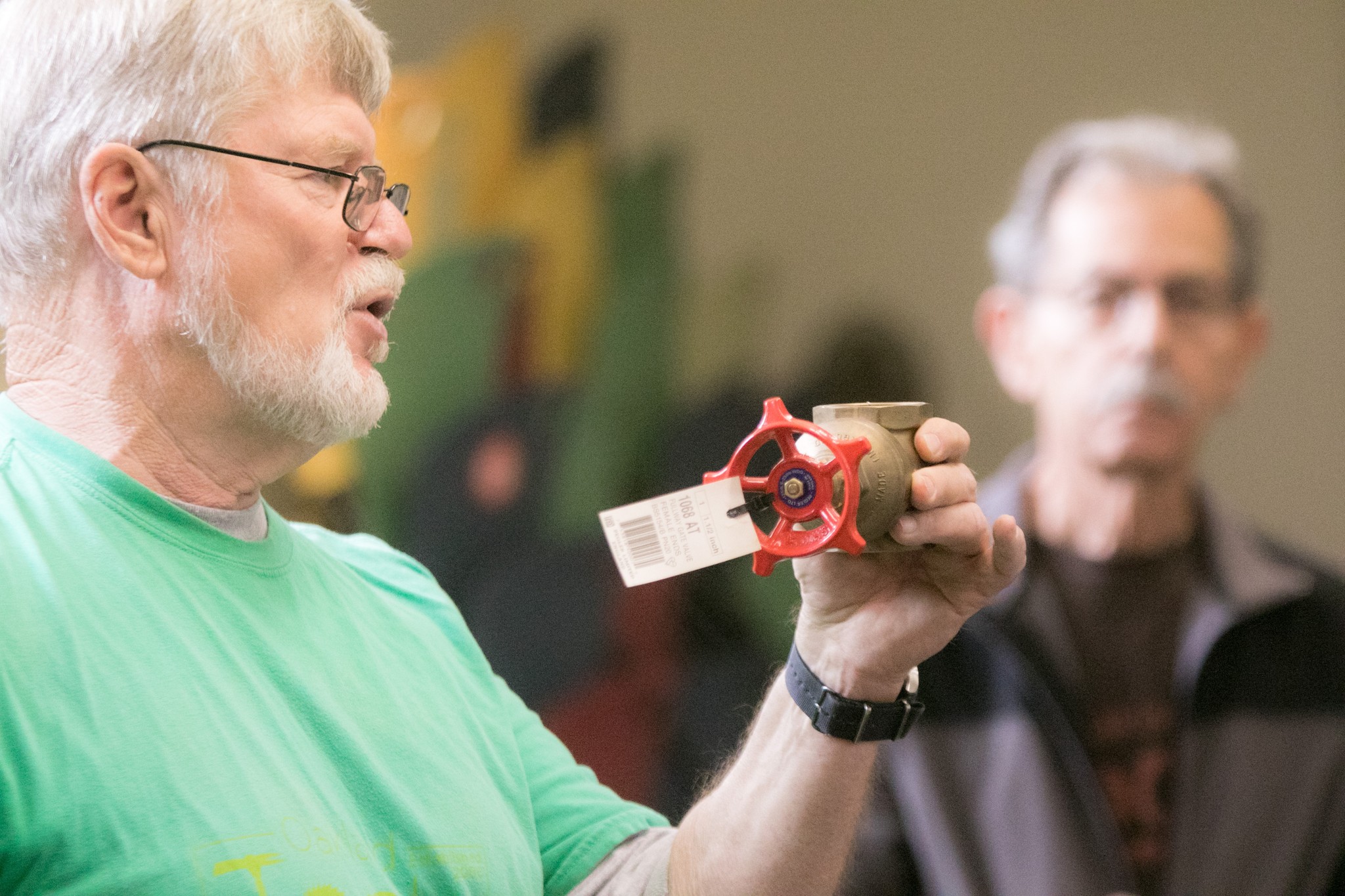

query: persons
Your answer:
[0,0,1025,896]
[829,106,1345,896]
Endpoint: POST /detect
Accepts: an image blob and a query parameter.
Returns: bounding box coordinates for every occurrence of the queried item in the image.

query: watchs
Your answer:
[787,638,925,749]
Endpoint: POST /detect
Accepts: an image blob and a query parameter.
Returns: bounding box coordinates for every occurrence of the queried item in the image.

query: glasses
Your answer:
[136,139,411,233]
